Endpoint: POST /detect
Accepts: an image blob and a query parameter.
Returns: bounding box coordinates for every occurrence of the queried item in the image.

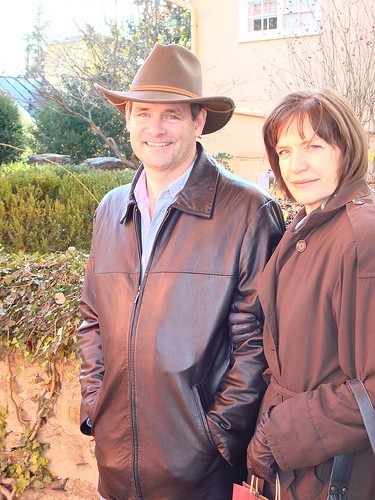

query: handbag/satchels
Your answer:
[232,471,280,500]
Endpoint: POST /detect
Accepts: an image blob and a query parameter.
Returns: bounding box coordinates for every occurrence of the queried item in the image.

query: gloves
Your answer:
[246,405,284,484]
[228,303,261,349]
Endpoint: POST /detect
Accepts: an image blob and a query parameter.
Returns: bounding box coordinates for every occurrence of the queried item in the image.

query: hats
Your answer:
[94,42,236,135]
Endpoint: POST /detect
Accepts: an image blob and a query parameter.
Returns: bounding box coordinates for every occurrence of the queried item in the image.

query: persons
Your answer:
[76,43,287,500]
[247,87,375,500]
